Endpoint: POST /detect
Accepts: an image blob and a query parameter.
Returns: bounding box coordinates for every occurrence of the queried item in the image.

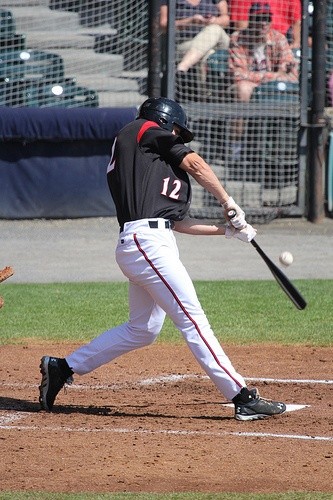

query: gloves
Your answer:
[220,197,247,231]
[224,220,257,243]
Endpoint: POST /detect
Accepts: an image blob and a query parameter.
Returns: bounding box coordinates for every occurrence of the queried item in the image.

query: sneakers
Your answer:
[39,355,72,412]
[234,388,287,421]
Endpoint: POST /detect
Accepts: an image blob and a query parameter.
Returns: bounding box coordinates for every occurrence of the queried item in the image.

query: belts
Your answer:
[120,219,176,233]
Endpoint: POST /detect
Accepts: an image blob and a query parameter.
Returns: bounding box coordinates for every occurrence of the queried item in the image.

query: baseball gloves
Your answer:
[0,266,14,308]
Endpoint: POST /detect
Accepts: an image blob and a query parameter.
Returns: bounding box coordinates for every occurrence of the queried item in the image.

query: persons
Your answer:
[39,98,287,421]
[160,0,333,163]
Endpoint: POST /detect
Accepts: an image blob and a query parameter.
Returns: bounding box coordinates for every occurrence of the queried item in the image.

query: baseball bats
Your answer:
[226,209,307,311]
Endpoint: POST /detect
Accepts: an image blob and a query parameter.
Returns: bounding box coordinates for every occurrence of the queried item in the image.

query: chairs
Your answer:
[247,81,300,171]
[0,11,98,108]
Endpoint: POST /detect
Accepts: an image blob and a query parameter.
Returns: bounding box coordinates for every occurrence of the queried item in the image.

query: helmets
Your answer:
[139,97,194,144]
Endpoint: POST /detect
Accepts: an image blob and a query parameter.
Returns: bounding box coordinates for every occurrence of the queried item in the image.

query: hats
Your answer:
[249,3,272,17]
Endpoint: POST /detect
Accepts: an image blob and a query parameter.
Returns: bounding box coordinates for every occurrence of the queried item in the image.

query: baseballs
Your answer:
[280,252,293,265]
